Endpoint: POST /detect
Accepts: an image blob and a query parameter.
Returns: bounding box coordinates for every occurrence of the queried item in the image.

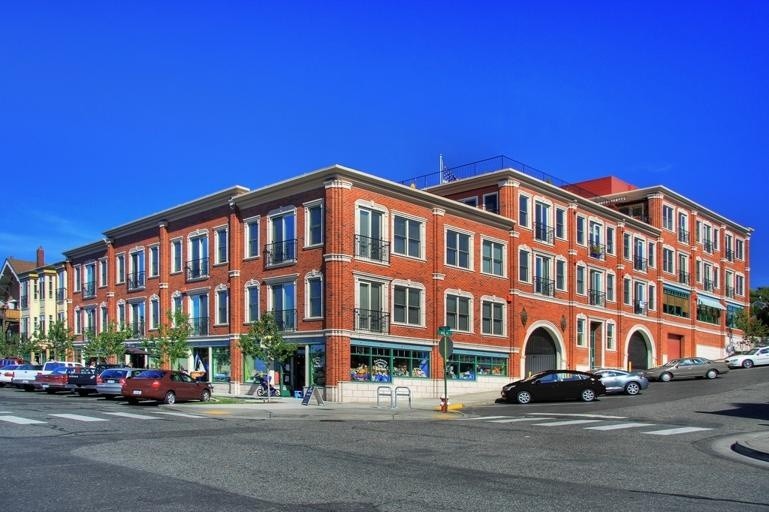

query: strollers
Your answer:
[254,374,283,399]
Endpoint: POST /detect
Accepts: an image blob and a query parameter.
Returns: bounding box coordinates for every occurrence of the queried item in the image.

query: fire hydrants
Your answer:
[438,393,453,415]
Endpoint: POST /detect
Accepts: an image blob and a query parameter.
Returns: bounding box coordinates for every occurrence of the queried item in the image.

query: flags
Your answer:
[440,164,460,184]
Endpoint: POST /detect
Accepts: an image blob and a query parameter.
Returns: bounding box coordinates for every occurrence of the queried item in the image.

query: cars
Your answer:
[726,345,769,370]
[0,355,213,409]
[641,356,731,386]
[501,369,608,406]
[584,367,651,397]
